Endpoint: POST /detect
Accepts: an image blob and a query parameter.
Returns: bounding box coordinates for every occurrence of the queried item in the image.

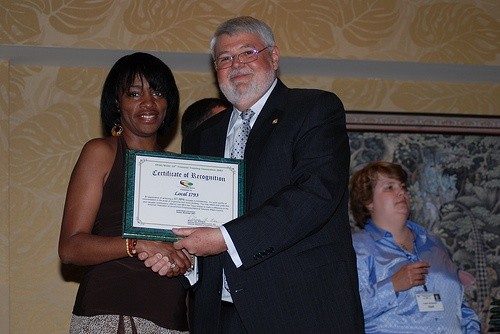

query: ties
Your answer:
[232,108,255,159]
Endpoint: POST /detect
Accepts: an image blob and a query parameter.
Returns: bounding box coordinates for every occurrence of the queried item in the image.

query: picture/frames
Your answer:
[346,110,500,334]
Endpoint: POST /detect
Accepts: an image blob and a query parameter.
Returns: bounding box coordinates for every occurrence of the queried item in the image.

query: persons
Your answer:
[351,162,481,334]
[138,16,366,334]
[58,52,194,334]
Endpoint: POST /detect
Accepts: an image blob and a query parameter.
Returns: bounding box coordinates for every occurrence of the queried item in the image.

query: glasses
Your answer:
[215,45,271,70]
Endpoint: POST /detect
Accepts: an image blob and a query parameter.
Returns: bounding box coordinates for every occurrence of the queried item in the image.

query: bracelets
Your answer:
[125,239,133,258]
[133,238,137,254]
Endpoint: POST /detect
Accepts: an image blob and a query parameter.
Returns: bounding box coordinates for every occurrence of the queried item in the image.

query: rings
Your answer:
[171,262,176,268]
